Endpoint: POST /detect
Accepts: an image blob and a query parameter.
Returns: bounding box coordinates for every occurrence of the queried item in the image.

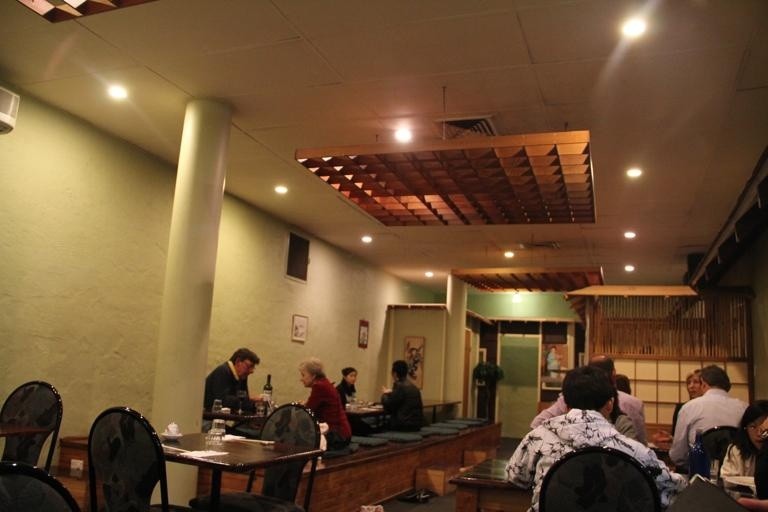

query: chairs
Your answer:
[702,425,739,457]
[189,402,321,512]
[0,460,81,512]
[0,380,62,472]
[539,446,660,512]
[88,406,198,512]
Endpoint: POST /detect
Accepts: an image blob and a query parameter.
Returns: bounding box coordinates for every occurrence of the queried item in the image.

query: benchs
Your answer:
[198,416,502,512]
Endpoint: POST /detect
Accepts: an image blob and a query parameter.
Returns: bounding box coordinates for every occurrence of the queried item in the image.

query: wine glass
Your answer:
[236,391,246,414]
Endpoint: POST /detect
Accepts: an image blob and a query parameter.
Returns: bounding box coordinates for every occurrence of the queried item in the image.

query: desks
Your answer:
[159,431,323,512]
[203,393,462,438]
[448,457,531,512]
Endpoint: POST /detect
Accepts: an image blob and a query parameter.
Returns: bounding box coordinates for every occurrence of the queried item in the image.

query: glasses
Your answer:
[243,360,255,370]
[745,426,765,433]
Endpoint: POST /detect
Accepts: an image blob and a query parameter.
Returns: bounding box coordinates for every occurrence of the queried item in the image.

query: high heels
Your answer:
[417,489,435,499]
[399,490,430,504]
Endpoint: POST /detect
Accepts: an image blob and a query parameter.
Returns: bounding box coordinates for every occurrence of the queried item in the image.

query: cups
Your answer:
[349,397,358,412]
[259,394,271,402]
[207,430,223,451]
[255,403,264,415]
[167,423,177,435]
[362,400,369,408]
[211,400,223,415]
[211,420,225,440]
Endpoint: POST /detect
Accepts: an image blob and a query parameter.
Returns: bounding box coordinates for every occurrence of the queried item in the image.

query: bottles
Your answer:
[263,374,272,408]
[687,428,710,481]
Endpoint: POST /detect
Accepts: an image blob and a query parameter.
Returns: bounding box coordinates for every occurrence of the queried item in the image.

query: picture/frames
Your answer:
[404,336,424,390]
[291,314,307,343]
[359,320,369,348]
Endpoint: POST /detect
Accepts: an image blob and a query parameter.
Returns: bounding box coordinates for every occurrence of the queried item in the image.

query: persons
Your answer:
[380,361,423,433]
[616,373,632,394]
[529,355,647,440]
[203,348,261,414]
[298,358,352,452]
[600,390,638,442]
[505,366,688,512]
[335,367,374,437]
[672,369,702,436]
[667,363,748,467]
[720,399,768,511]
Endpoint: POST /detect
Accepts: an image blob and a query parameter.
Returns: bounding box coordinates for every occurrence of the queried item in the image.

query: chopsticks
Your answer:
[238,438,273,446]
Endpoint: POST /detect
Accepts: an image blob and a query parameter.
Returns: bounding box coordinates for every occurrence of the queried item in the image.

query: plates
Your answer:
[159,434,182,441]
